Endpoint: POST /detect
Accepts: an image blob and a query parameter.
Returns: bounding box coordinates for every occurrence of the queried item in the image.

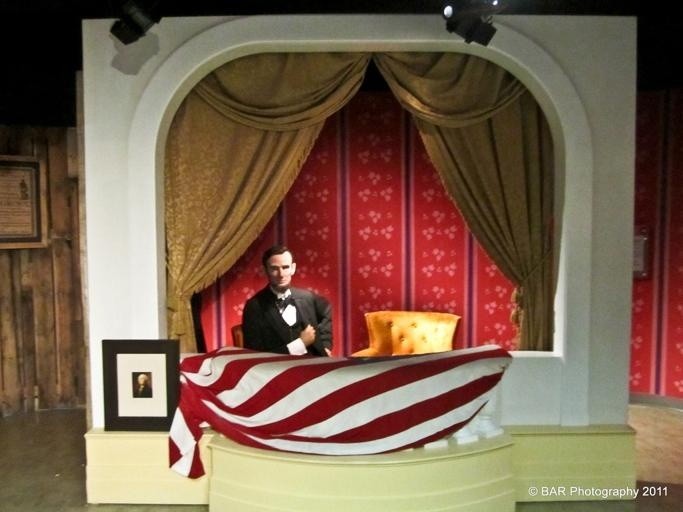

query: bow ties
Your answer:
[278,296,295,310]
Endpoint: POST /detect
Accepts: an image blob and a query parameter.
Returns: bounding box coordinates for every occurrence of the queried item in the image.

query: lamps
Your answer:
[439,0,508,47]
[110,0,166,45]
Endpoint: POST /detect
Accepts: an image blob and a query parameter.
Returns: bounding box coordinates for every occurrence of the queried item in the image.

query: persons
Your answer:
[135,374,151,397]
[243,246,333,357]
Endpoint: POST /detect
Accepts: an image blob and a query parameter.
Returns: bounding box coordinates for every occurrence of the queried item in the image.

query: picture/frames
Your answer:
[101,339,181,430]
[633,224,653,281]
[0,154,48,250]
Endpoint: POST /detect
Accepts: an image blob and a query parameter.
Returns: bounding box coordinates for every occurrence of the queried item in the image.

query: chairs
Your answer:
[231,324,246,350]
[348,311,462,357]
